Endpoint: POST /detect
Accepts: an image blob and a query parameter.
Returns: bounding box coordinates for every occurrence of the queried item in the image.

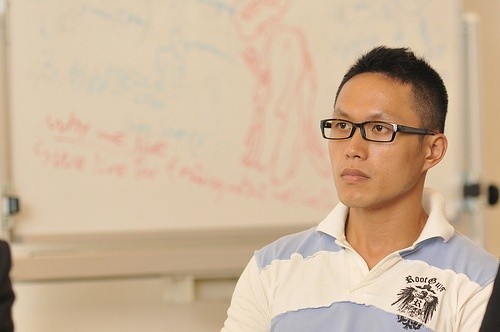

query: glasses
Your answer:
[321,119,434,143]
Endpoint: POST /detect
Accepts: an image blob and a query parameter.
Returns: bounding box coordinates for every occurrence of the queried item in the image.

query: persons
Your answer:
[213,44,499,331]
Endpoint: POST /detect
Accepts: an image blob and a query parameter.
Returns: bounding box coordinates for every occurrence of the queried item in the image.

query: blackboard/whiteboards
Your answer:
[1,0,476,283]
